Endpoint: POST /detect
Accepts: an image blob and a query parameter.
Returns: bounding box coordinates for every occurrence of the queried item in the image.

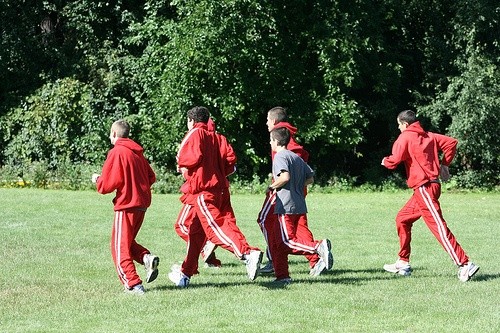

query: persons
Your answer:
[257,107,326,276]
[91,119,160,296]
[167,107,263,286]
[268,127,334,282]
[380,110,480,282]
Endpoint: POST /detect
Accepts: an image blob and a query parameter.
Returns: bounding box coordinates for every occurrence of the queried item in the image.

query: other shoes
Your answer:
[129,283,146,294]
[202,262,219,268]
[260,261,274,274]
[202,241,218,263]
[143,253,159,283]
[167,269,189,287]
[383,259,410,277]
[308,257,325,276]
[317,238,334,270]
[243,249,263,280]
[458,261,480,282]
